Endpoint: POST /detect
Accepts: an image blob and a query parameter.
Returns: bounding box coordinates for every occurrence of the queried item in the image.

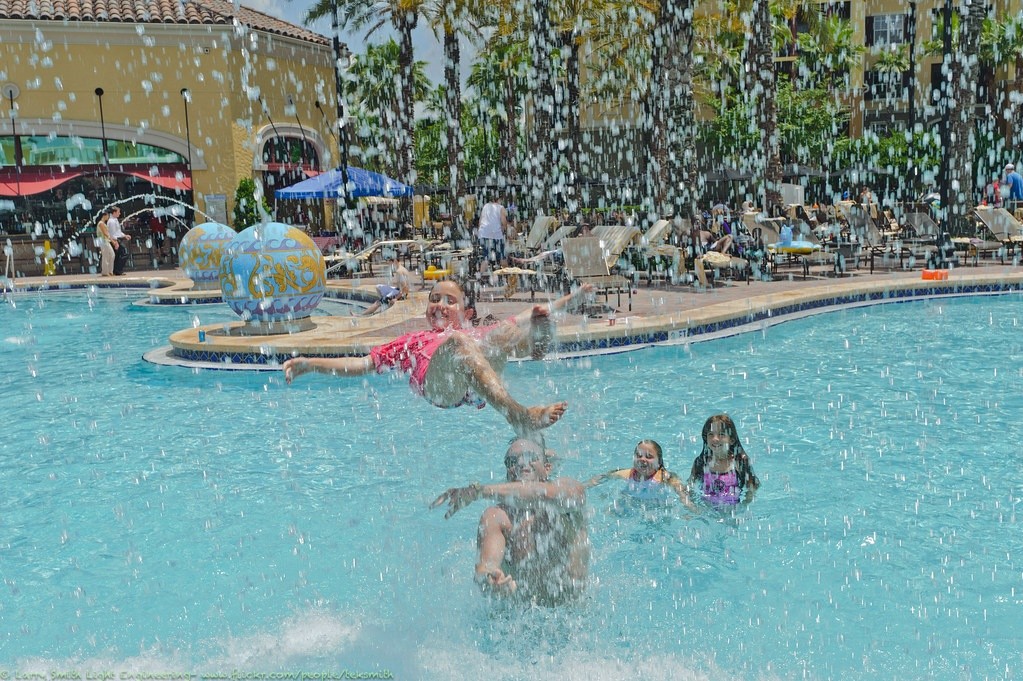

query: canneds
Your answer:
[199,329,205,342]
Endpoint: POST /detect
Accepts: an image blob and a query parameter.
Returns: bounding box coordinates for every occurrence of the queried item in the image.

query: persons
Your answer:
[860,185,873,204]
[879,205,896,230]
[583,440,700,514]
[687,414,762,503]
[361,256,410,315]
[983,164,1023,215]
[429,431,593,606]
[97,206,132,276]
[151,212,171,265]
[399,203,817,290]
[478,194,508,285]
[283,277,598,436]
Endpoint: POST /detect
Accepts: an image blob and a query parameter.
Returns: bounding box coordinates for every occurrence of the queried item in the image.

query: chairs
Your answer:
[128,233,156,269]
[309,201,1023,315]
[57,234,86,274]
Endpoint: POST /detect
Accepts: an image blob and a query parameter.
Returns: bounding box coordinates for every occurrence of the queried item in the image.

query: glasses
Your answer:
[504,451,545,468]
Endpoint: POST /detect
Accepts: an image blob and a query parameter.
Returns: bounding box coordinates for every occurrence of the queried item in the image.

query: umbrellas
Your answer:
[411,162,887,196]
[275,166,415,200]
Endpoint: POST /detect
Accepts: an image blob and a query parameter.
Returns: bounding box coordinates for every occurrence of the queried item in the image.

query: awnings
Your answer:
[0,163,192,196]
